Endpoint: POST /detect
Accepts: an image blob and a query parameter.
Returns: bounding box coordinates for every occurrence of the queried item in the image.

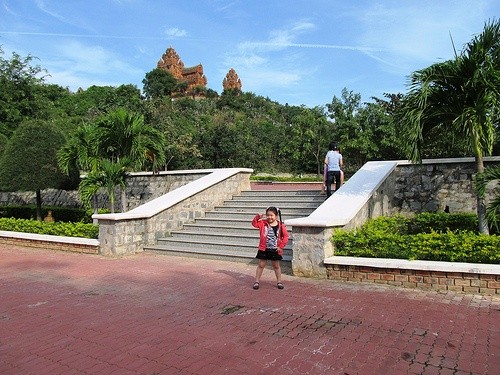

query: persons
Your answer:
[321,142,344,200]
[252,207,288,290]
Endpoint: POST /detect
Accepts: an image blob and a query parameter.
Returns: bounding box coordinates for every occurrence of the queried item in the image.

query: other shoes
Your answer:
[253,281,259,289]
[277,281,284,288]
